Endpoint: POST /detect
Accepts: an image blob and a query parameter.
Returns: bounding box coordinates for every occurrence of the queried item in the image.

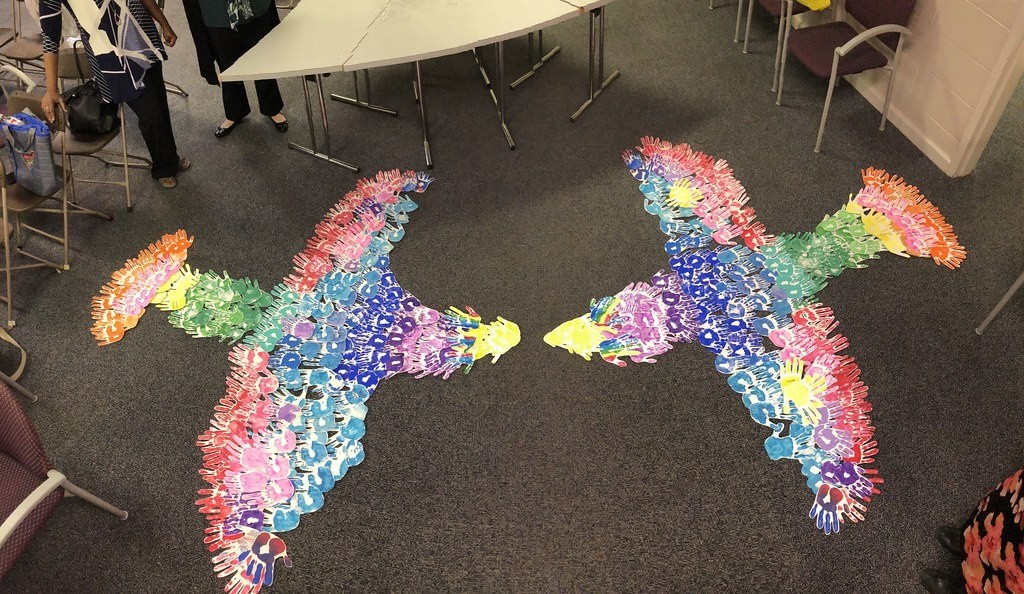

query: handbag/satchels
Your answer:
[61,79,121,135]
[0,108,56,197]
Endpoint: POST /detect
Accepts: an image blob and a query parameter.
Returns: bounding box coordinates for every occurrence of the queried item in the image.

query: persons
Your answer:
[39,0,191,190]
[921,468,1024,594]
[183,0,288,139]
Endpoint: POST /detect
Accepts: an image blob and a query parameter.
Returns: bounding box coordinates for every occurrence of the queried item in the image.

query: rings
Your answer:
[45,107,50,111]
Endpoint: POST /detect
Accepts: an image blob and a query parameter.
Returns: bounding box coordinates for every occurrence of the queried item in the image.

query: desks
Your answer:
[219,0,397,173]
[510,0,620,124]
[342,0,582,170]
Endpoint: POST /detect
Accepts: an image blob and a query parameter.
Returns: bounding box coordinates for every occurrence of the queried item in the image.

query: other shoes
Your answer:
[179,157,190,170]
[270,113,288,132]
[159,177,177,187]
[215,119,242,138]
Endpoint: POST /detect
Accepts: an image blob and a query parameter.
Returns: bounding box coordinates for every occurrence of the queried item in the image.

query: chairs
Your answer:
[742,0,838,93]
[775,0,915,154]
[0,0,190,404]
[0,384,131,582]
[708,0,743,43]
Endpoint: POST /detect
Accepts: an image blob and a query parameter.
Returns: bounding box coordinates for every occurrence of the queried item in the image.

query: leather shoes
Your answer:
[936,526,967,556]
[920,568,967,594]
